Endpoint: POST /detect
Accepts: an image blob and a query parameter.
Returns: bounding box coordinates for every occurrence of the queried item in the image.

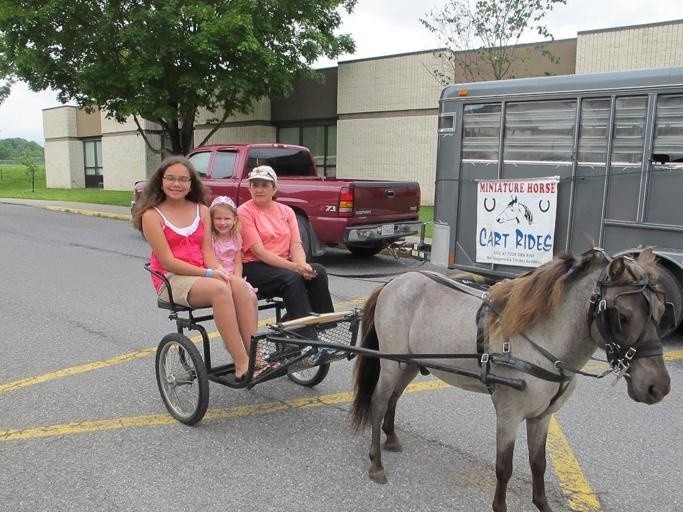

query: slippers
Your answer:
[234,360,271,382]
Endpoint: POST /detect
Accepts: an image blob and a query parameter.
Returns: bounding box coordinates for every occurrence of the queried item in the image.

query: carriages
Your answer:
[143,244,670,512]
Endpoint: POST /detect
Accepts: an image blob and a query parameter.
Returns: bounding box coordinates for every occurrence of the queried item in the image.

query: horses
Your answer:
[346,245,672,512]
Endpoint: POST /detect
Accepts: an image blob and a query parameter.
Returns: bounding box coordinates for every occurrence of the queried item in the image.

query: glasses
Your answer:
[163,176,191,182]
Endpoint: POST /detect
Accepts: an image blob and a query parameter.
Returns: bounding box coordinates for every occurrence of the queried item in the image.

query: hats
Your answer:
[246,165,277,180]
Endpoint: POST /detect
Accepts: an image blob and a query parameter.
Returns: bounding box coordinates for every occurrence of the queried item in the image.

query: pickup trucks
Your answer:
[130,143,422,263]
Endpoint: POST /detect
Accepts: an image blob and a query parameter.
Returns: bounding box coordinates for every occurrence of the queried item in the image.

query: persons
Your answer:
[209,194,259,296]
[130,156,282,382]
[236,164,348,364]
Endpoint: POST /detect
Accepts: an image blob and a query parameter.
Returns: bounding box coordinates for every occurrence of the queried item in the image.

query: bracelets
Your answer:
[205,268,212,277]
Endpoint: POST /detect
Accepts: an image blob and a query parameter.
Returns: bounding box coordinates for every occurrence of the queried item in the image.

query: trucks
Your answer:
[430,65,683,342]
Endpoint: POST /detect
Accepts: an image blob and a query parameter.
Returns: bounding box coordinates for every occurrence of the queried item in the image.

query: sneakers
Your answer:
[301,346,329,367]
[318,346,346,361]
